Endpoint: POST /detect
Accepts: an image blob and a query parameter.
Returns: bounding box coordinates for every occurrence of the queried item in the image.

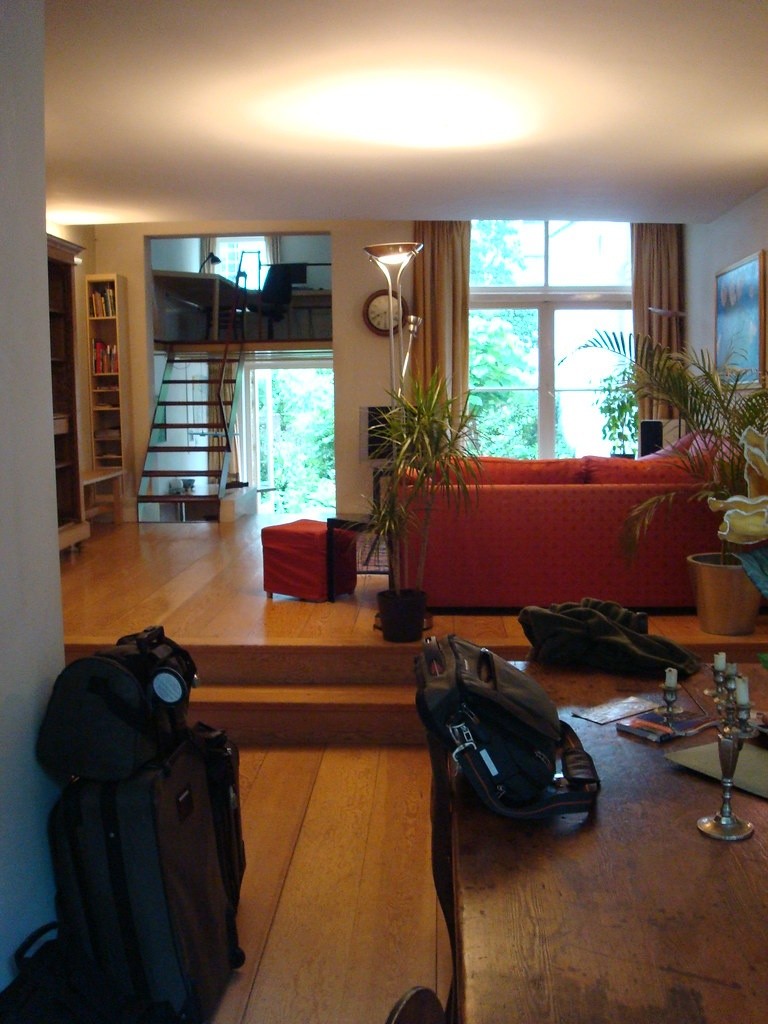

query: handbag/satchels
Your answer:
[0,920,178,1024]
[414,632,601,819]
[36,626,197,807]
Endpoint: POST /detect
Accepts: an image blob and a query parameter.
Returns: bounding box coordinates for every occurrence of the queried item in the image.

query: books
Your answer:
[88,283,118,439]
[616,705,721,744]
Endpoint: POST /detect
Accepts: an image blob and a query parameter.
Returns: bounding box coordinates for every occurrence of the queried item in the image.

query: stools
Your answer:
[261,520,358,603]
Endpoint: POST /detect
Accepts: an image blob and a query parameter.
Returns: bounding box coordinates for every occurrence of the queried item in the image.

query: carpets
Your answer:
[356,534,392,575]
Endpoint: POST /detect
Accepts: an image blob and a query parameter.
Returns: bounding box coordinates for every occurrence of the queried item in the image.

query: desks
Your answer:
[152,269,247,341]
[432,660,768,1024]
[287,288,332,340]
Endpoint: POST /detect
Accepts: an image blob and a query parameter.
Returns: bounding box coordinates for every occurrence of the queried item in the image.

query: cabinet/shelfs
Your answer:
[85,274,134,496]
[34,233,91,555]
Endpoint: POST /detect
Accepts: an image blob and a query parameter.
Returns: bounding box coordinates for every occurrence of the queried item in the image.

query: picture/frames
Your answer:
[712,248,765,389]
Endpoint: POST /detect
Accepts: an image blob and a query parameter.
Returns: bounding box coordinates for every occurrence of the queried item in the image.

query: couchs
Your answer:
[391,428,746,612]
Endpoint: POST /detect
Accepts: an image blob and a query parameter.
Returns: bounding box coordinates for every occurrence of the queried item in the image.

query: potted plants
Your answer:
[581,327,768,637]
[361,362,482,642]
[593,362,644,460]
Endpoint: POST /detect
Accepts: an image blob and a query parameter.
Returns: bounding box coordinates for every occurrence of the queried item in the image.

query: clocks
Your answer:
[362,290,409,337]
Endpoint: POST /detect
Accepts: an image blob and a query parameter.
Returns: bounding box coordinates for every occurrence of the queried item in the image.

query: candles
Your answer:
[725,661,738,677]
[666,666,678,689]
[733,670,749,706]
[714,651,726,670]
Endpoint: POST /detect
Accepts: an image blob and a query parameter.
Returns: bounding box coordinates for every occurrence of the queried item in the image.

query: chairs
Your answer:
[243,266,293,340]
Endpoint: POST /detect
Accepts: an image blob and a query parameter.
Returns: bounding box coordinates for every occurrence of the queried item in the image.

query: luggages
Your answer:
[51,720,246,1023]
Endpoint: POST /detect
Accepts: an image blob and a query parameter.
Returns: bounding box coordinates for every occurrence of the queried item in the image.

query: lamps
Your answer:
[364,240,438,631]
[198,252,220,274]
